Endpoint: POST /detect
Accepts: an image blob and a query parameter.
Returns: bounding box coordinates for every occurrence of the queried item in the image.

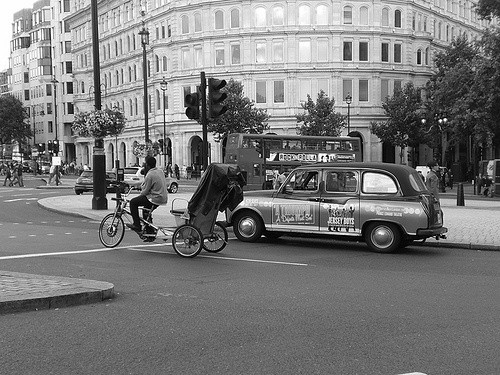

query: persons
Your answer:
[174,164,180,181]
[61,160,84,176]
[418,171,425,182]
[426,160,448,240]
[435,167,454,193]
[182,163,193,180]
[168,164,172,178]
[476,173,492,197]
[0,159,26,188]
[272,169,286,189]
[126,157,167,242]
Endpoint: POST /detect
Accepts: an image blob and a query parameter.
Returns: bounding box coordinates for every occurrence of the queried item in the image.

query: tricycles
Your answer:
[99,162,247,258]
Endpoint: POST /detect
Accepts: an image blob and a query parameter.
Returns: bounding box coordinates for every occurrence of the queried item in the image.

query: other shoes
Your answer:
[2,183,25,187]
[47,184,51,185]
[126,223,141,232]
[60,181,62,183]
[58,183,60,184]
[143,237,155,242]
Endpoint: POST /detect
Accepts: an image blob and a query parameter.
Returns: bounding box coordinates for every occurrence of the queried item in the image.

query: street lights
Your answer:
[346,91,353,134]
[50,66,60,141]
[159,76,168,166]
[138,24,150,144]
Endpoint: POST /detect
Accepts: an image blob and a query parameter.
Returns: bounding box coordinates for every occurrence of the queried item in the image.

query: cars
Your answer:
[111,166,179,194]
[21,160,52,173]
[412,166,454,192]
[226,162,448,254]
[75,170,130,195]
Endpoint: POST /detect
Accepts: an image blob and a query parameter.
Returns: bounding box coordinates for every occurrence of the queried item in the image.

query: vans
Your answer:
[478,158,500,197]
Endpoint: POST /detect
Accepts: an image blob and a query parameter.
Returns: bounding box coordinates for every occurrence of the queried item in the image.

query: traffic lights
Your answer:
[184,93,200,119]
[208,77,229,118]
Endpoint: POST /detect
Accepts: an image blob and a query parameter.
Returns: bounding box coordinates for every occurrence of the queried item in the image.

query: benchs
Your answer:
[169,197,189,216]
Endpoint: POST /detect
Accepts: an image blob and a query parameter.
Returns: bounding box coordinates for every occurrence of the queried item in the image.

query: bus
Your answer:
[220,132,362,190]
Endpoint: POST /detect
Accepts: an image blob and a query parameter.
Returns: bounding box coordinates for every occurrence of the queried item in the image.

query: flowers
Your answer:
[130,141,162,158]
[71,109,128,139]
